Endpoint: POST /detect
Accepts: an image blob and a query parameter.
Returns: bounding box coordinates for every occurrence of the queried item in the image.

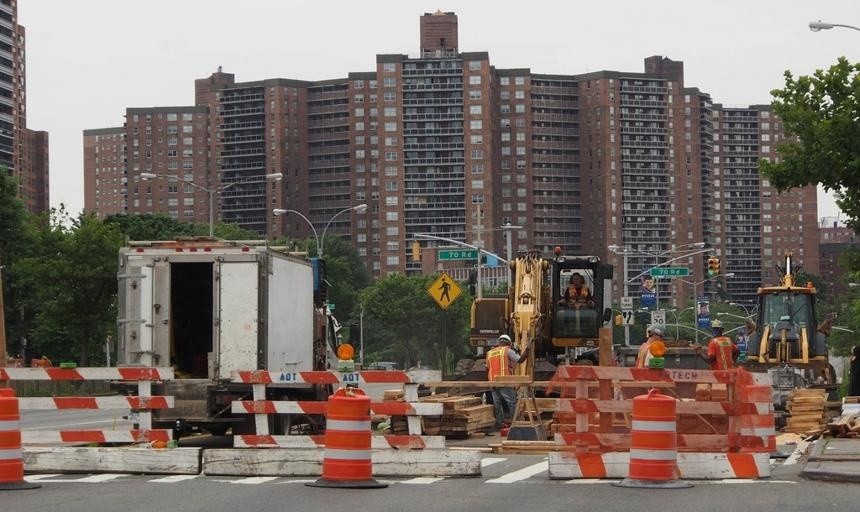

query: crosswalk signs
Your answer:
[426,272,462,311]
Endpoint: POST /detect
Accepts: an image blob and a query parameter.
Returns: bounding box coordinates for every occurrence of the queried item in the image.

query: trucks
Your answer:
[116,237,366,435]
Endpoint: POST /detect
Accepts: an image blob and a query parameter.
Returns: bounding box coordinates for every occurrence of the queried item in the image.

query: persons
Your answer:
[486,334,533,429]
[696,319,740,370]
[557,273,595,309]
[635,322,665,368]
[848,346,860,396]
[698,302,709,318]
[642,275,656,294]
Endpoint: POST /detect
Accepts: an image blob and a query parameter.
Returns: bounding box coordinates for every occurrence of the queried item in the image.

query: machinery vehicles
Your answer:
[454,246,614,375]
[746,252,836,388]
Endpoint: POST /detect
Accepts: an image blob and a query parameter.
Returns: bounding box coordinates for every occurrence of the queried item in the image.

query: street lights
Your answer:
[139,172,284,237]
[273,202,369,260]
[607,241,758,346]
[809,17,860,37]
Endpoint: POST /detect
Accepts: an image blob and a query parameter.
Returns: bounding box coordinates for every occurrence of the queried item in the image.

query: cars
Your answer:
[368,361,398,370]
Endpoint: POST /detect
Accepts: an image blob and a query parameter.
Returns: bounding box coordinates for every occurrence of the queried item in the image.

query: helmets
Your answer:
[649,324,664,336]
[498,334,511,342]
[712,319,724,328]
